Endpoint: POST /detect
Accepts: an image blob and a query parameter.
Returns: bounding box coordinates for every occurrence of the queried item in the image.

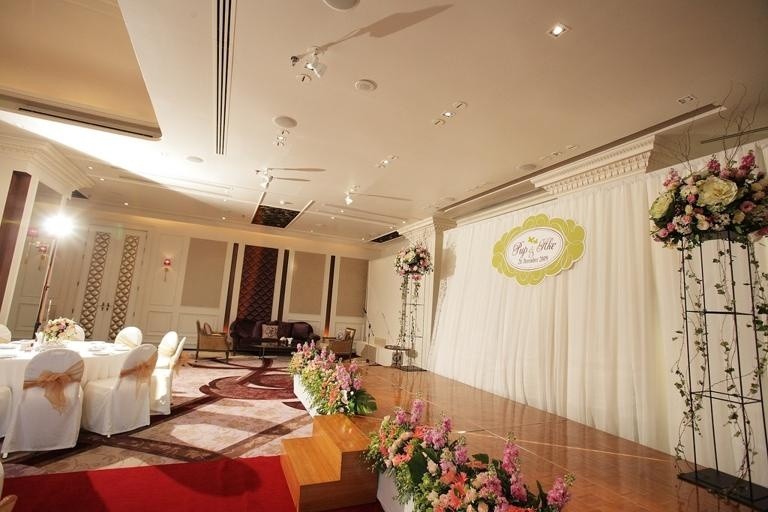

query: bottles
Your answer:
[280,336,286,346]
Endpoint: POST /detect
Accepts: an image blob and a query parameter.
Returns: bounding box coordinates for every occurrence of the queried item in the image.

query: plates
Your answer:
[1,340,129,361]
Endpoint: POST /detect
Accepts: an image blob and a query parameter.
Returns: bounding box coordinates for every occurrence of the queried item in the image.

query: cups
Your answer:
[287,338,294,346]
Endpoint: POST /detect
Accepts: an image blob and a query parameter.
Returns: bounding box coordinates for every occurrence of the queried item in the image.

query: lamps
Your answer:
[306,49,328,78]
[164,259,170,281]
[25,228,47,264]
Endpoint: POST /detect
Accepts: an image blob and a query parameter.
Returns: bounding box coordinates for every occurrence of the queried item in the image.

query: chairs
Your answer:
[317,328,356,362]
[197,321,229,362]
[0,323,186,458]
[374,337,386,347]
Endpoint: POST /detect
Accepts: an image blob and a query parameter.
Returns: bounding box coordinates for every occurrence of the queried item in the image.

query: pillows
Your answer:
[336,330,350,340]
[204,322,212,335]
[262,324,278,338]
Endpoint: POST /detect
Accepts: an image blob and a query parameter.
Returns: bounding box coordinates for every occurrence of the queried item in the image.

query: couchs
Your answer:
[229,319,320,356]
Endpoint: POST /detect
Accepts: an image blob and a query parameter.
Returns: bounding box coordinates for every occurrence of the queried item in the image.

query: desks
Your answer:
[356,341,408,366]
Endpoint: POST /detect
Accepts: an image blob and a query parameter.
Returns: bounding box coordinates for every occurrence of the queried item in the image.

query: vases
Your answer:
[294,375,330,418]
[377,472,414,512]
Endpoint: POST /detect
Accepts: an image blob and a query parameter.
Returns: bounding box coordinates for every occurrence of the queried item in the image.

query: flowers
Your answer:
[362,392,574,512]
[291,340,377,417]
[394,244,434,280]
[650,151,768,249]
